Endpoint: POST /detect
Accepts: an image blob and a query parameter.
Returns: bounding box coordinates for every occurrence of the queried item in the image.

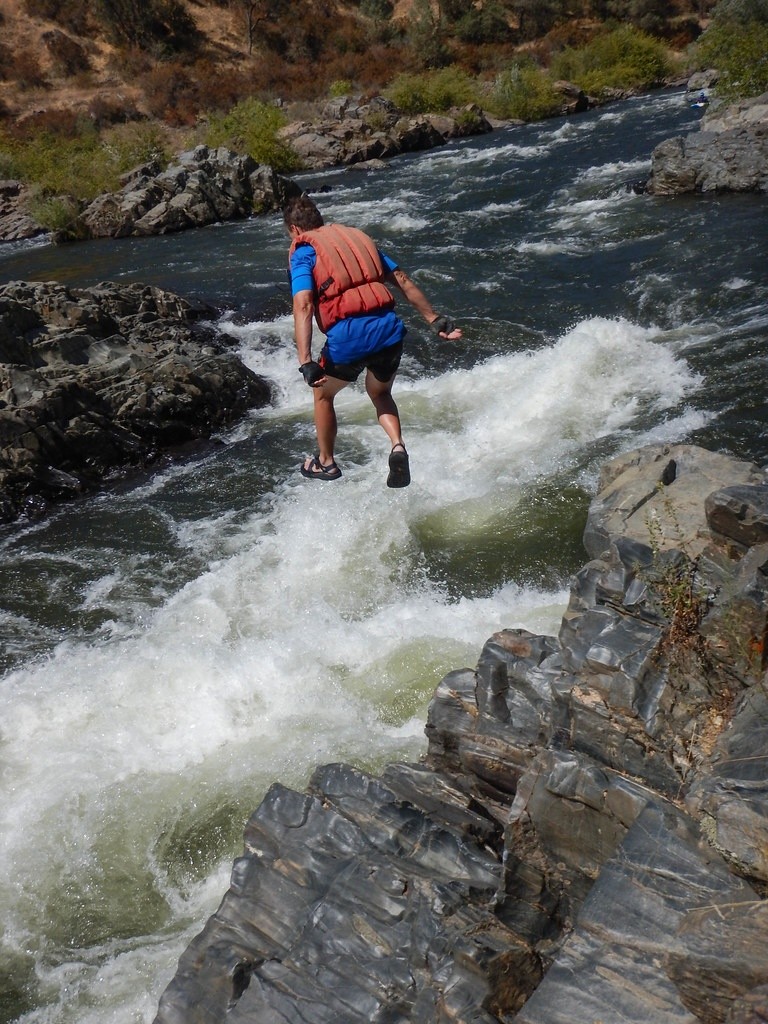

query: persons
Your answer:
[279,191,464,490]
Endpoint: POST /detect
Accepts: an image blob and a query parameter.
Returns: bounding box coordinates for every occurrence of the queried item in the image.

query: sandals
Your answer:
[386,443,410,488]
[301,454,342,480]
